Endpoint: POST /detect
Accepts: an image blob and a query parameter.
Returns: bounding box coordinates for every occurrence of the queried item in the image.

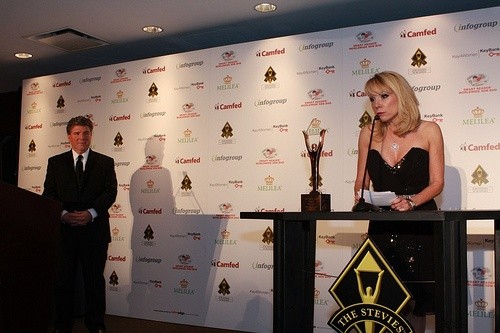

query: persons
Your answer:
[42,116,119,333]
[353,71,445,333]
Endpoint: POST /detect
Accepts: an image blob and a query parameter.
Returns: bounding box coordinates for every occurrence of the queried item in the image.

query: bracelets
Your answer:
[406,198,416,209]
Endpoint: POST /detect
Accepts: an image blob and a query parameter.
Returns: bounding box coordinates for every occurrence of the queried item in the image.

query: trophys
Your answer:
[300,129,331,211]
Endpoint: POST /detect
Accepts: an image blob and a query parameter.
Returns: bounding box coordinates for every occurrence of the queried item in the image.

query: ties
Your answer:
[76,155,84,195]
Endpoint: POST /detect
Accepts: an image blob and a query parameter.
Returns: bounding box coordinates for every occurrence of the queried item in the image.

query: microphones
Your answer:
[351,115,380,212]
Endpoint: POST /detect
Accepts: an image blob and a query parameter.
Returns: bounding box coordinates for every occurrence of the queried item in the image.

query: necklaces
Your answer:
[386,125,424,151]
